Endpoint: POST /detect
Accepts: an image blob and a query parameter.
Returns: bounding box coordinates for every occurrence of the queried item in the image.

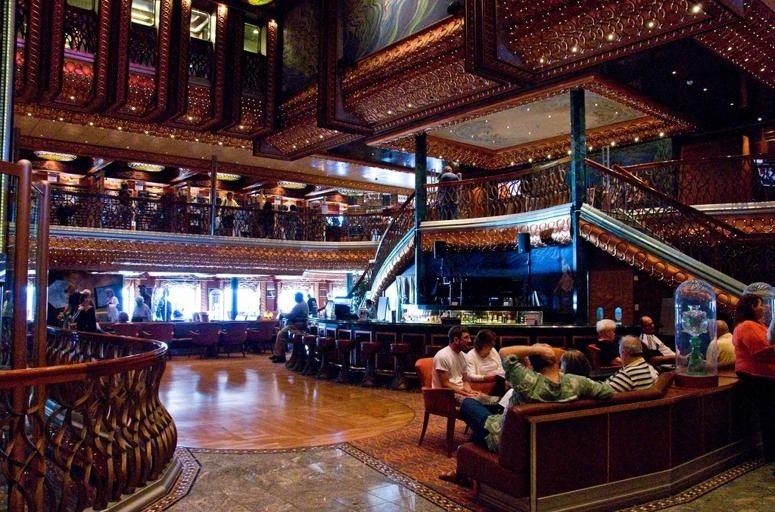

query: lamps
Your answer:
[277,179,307,190]
[33,150,78,162]
[126,162,165,172]
[207,170,241,182]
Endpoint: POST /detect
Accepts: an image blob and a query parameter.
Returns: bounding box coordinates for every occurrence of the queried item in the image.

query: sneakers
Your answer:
[269,355,286,363]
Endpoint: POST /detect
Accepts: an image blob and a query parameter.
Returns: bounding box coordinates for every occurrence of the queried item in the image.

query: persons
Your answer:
[118,182,130,230]
[158,186,182,232]
[58,286,172,358]
[433,319,661,486]
[438,165,461,220]
[217,193,242,235]
[268,292,377,363]
[638,295,775,462]
[261,197,275,237]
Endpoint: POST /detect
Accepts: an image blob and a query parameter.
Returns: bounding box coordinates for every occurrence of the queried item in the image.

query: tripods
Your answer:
[518,253,546,306]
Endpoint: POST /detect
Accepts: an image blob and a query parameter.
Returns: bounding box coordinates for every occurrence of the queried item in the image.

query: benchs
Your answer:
[458,350,775,511]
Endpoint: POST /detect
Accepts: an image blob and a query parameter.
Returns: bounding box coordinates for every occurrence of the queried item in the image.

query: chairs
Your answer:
[586,343,621,371]
[99,320,277,360]
[413,358,470,459]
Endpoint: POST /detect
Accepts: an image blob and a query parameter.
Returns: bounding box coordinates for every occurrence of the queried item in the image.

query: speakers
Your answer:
[517,232,531,254]
[433,241,446,260]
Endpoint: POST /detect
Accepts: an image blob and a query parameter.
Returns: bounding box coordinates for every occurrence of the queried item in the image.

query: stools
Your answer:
[284,328,443,392]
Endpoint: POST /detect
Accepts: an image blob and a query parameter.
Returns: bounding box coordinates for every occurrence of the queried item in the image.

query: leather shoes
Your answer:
[439,470,456,481]
